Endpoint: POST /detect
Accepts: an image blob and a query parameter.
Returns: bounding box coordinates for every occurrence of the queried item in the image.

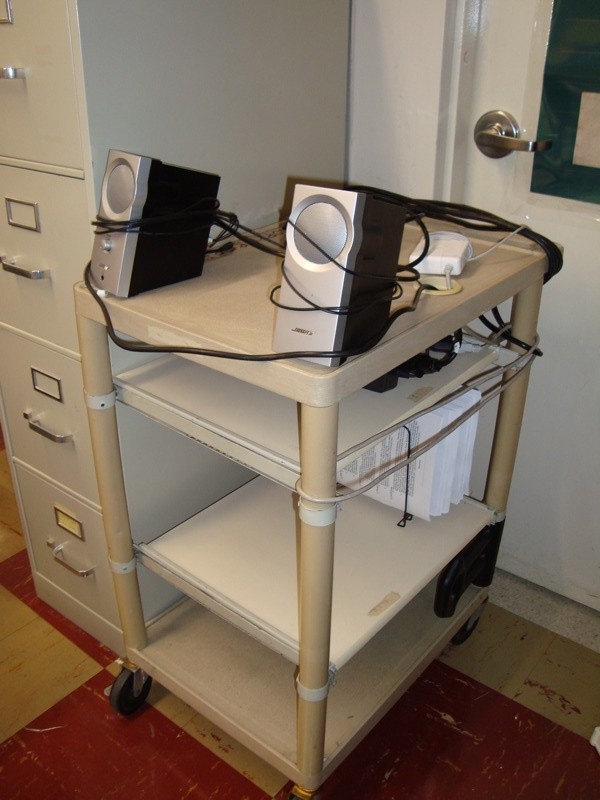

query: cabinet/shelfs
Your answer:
[0,0,350,659]
[73,219,543,800]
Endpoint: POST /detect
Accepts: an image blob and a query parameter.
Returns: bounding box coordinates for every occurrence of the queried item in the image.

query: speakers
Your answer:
[87,147,222,299]
[269,183,407,367]
